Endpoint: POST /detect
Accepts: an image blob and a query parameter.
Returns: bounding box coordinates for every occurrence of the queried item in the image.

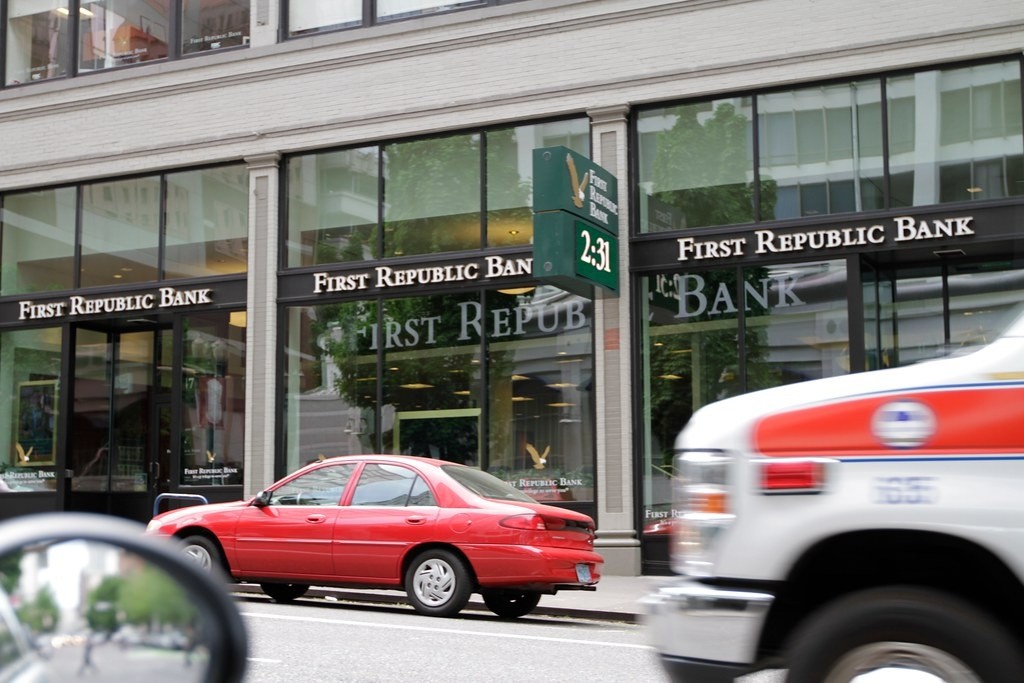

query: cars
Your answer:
[147,453,605,621]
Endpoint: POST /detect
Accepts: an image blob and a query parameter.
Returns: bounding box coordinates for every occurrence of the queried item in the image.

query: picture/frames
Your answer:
[14,380,60,467]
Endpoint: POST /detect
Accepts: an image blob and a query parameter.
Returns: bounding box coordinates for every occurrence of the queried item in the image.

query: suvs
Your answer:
[639,303,1024,683]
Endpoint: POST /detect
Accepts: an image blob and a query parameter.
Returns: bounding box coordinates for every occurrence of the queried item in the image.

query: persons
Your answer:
[77,635,99,676]
[184,627,195,667]
